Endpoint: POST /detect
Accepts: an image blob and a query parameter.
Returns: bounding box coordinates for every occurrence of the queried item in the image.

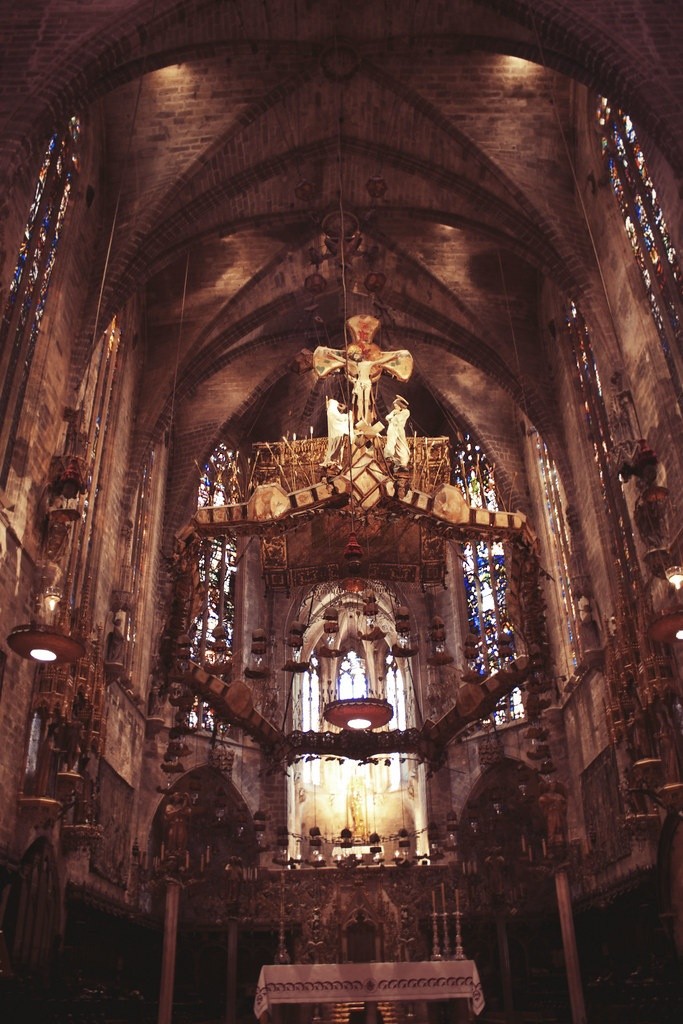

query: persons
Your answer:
[539,782,567,842]
[330,348,399,422]
[51,720,85,773]
[166,792,193,856]
[385,399,411,470]
[110,619,124,660]
[324,400,352,467]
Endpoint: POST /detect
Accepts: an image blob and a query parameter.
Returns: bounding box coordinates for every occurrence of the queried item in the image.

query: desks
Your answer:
[255,960,485,1024]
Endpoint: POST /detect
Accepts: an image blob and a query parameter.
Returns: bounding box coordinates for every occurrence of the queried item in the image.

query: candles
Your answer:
[431,890,435,913]
[441,883,446,914]
[455,889,460,912]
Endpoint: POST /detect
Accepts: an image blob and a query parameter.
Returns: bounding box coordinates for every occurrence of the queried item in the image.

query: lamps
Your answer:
[6,0,683,732]
